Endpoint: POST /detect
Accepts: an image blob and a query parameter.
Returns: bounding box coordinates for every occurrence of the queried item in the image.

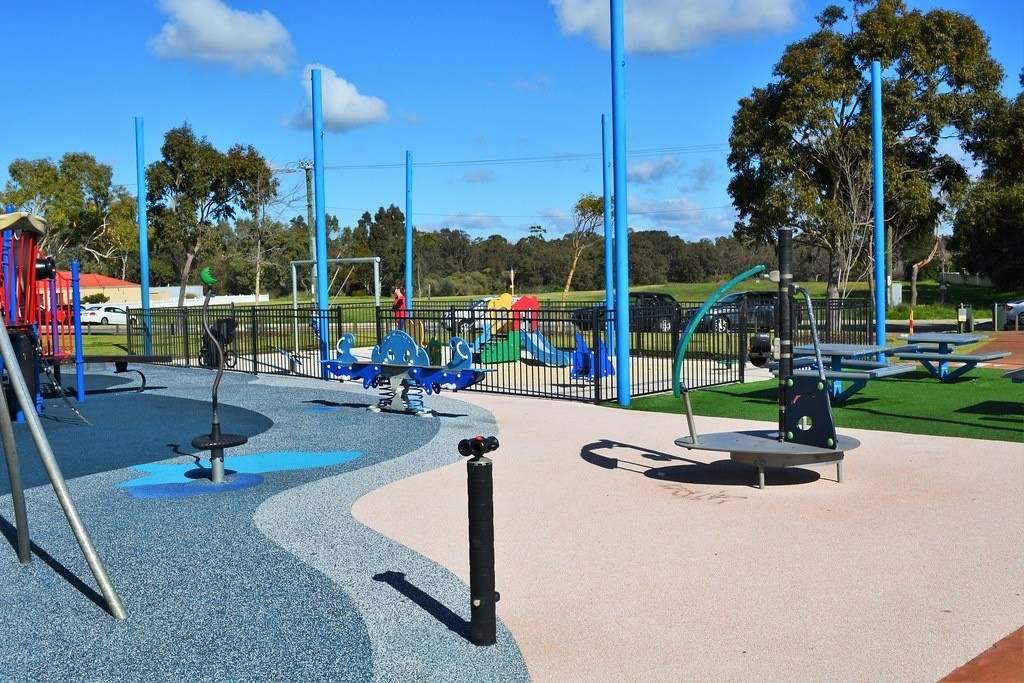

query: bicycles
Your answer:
[198,332,237,368]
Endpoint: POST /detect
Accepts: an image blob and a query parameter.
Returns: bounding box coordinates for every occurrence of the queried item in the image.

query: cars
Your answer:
[80,304,139,325]
[0,302,87,325]
[1006,299,1024,325]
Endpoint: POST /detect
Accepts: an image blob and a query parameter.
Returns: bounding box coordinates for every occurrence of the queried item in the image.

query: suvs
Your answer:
[684,290,803,333]
[568,291,683,331]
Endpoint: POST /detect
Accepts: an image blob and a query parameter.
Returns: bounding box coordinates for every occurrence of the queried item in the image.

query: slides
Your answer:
[519,329,574,367]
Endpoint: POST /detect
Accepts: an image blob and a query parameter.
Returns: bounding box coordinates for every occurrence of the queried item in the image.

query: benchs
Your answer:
[768,345,1011,403]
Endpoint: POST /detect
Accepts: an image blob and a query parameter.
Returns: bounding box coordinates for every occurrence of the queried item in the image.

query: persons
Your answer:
[755,274,760,285]
[391,285,406,330]
[308,308,320,339]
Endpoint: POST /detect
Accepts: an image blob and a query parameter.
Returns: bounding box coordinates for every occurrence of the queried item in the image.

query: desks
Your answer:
[897,333,990,379]
[793,344,891,399]
